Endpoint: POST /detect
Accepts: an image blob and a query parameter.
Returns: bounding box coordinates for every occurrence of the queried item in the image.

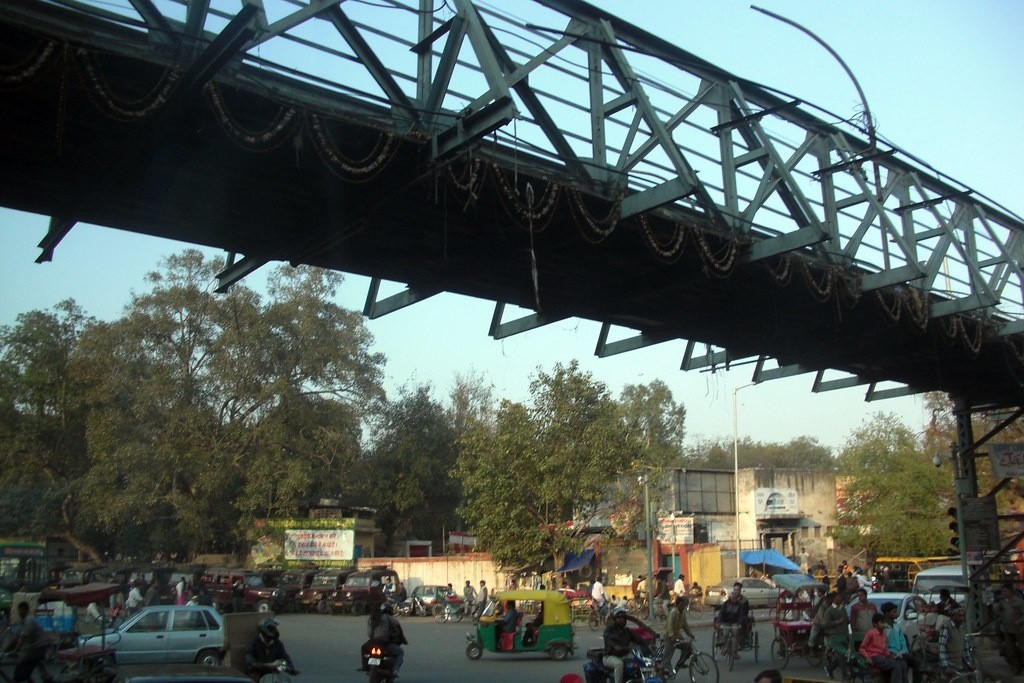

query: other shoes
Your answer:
[392,671,399,678]
[676,662,688,668]
[360,667,367,673]
[721,649,727,656]
[824,665,834,679]
[733,652,741,660]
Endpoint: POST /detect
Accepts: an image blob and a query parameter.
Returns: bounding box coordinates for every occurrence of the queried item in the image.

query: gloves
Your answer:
[615,645,623,653]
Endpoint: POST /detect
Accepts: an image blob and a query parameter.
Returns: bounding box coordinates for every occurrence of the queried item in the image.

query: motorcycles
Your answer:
[582,643,667,683]
[364,639,409,683]
[386,592,430,618]
[465,588,580,660]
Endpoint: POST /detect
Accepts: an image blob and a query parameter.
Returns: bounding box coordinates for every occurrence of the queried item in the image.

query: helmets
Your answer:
[611,607,628,627]
[381,602,393,612]
[257,617,279,640]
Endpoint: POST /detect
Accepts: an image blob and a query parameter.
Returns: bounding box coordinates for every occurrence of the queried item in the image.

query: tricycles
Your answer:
[0,580,122,683]
[712,605,760,671]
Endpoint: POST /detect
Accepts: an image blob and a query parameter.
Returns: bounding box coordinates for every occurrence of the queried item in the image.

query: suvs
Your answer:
[0,564,360,622]
[325,565,406,617]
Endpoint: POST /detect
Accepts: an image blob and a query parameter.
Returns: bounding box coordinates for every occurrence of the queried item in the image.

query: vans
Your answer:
[912,565,977,606]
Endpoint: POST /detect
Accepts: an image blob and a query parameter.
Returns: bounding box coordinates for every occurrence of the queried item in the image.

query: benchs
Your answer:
[629,628,659,648]
[777,602,814,632]
[55,645,115,658]
[526,622,543,642]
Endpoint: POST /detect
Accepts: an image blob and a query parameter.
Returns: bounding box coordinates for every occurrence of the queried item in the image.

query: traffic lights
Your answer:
[947,506,959,548]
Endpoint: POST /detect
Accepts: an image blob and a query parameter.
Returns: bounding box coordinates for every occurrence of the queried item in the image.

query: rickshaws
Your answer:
[431,589,594,624]
[769,573,1024,683]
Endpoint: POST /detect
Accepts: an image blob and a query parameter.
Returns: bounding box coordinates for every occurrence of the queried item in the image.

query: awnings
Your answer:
[740,549,801,574]
[556,549,595,573]
[512,553,555,571]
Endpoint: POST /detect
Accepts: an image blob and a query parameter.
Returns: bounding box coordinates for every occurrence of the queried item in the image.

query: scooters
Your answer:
[248,658,300,683]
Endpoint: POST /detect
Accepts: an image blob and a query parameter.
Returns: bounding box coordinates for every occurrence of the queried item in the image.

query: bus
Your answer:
[871,555,961,593]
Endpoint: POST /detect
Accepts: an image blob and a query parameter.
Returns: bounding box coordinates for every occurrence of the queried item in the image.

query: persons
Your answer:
[0,574,118,683]
[244,617,300,683]
[126,572,247,613]
[445,570,571,648]
[589,573,782,683]
[788,547,1024,682]
[361,578,409,677]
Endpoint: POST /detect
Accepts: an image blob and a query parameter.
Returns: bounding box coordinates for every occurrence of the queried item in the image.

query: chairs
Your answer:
[500,612,523,651]
[426,590,432,595]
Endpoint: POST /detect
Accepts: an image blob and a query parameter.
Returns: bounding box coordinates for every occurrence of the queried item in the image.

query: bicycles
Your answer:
[651,636,719,683]
[559,581,706,632]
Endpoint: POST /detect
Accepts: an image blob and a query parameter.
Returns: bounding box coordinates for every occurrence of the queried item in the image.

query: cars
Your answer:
[76,605,230,672]
[843,592,939,654]
[410,584,466,617]
[704,577,784,610]
